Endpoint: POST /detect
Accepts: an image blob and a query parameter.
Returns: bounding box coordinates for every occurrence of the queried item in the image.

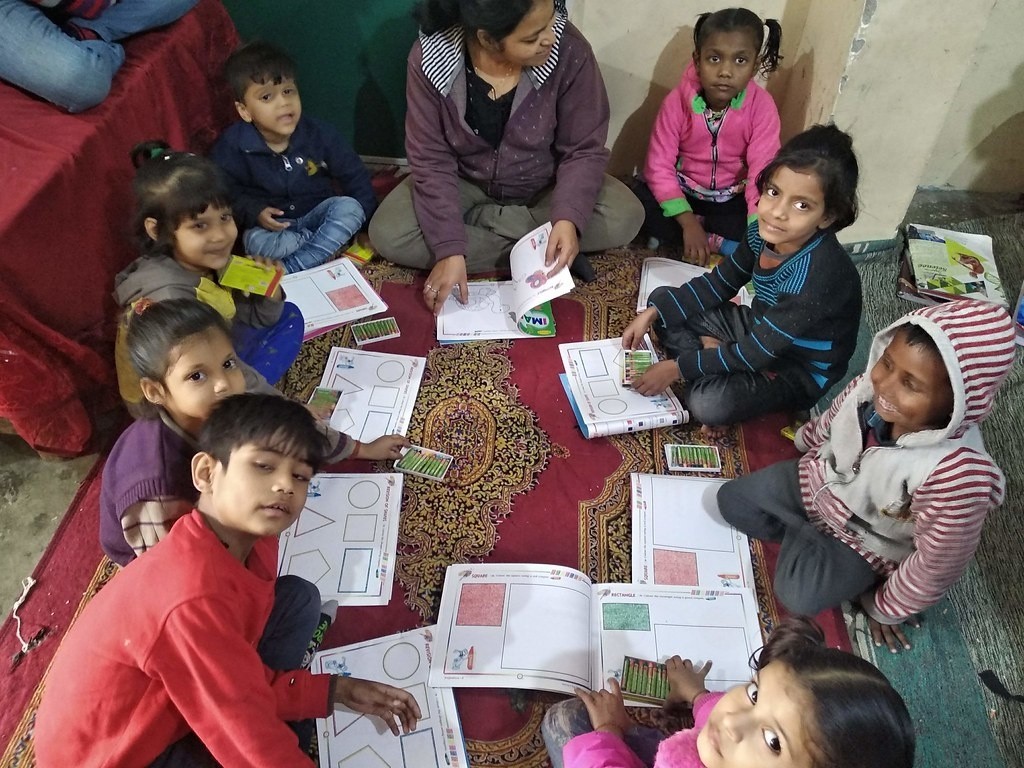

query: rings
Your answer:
[430,288,437,293]
[427,284,432,288]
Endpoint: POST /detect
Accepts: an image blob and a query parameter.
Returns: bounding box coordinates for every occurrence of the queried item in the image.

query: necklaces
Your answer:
[475,62,514,101]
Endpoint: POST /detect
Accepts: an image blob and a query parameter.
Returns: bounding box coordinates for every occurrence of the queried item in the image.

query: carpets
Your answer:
[0,177,852,768]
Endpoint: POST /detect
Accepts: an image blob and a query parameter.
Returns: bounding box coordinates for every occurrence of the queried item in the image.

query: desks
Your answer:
[1,0,244,464]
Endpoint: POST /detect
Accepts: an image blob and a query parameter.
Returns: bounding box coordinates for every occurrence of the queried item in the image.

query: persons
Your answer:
[202,39,377,274]
[97,296,411,575]
[540,613,915,768]
[716,300,1015,655]
[644,7,783,248]
[368,0,646,281]
[114,139,306,421]
[622,120,898,422]
[0,0,198,114]
[34,393,422,768]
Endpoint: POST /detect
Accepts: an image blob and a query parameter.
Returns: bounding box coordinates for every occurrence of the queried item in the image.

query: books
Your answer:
[558,332,691,438]
[276,468,762,768]
[636,257,716,314]
[273,253,426,444]
[433,218,576,345]
[905,218,1008,305]
